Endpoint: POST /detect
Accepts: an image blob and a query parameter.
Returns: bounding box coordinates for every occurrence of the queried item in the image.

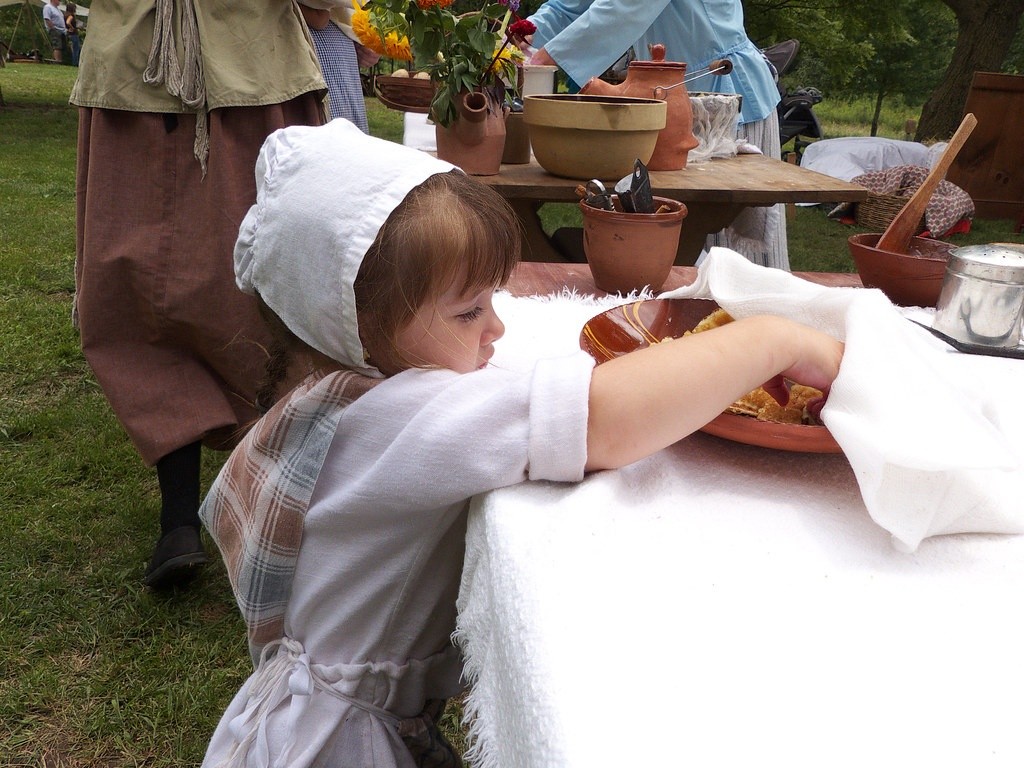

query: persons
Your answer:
[196,119,845,768]
[42,1,79,63]
[70,0,791,587]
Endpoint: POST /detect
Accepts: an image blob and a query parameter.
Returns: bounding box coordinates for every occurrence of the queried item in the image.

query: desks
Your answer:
[443,263,1024,768]
[403,110,867,266]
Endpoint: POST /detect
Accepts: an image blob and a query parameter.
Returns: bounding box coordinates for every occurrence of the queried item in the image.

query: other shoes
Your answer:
[143,526,209,586]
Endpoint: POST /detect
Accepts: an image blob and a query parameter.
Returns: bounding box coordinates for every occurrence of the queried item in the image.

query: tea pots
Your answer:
[578,42,733,173]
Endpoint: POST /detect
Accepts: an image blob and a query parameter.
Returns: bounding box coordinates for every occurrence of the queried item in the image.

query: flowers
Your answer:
[347,0,549,72]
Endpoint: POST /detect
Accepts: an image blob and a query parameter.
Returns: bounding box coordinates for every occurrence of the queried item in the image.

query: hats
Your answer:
[233,118,467,379]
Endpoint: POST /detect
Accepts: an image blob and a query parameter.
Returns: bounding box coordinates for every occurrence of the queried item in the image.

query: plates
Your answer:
[578,296,856,453]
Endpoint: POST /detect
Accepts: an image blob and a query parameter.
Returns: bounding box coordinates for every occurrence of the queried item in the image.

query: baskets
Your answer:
[851,174,927,237]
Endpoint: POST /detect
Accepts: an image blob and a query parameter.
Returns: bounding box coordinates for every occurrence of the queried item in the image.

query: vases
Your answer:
[579,195,688,298]
[437,85,508,176]
[524,93,667,179]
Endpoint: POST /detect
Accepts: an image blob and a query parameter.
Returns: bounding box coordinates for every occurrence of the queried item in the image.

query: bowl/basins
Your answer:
[522,91,668,181]
[687,91,742,145]
[847,230,963,310]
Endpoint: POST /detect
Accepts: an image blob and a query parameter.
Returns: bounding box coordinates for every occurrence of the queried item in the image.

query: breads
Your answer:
[658,307,824,427]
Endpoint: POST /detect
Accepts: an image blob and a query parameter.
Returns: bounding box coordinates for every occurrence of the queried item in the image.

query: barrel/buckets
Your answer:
[579,195,687,298]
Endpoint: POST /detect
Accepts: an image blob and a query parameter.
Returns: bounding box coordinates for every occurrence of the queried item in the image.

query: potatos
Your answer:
[391,69,431,80]
